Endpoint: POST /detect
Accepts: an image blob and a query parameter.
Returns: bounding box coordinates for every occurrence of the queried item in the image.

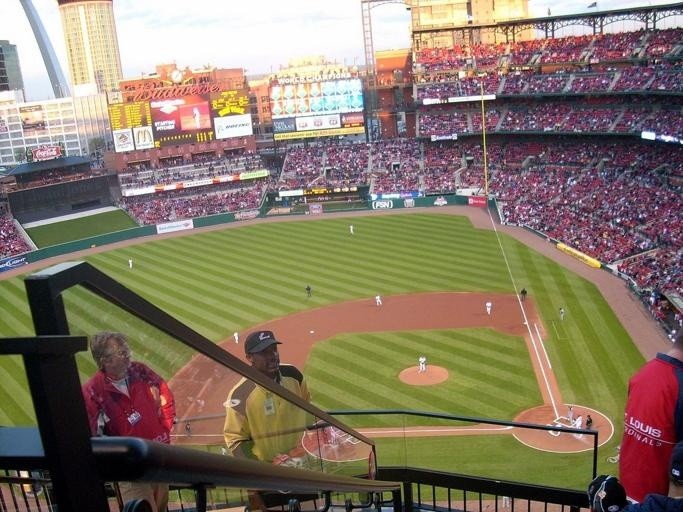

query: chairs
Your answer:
[525,219,682,347]
[409,28,682,227]
[116,138,418,226]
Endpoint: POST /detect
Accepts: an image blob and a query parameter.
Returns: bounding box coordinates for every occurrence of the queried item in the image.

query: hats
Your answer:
[243,330,283,354]
[670,441,683,480]
[586,474,626,512]
